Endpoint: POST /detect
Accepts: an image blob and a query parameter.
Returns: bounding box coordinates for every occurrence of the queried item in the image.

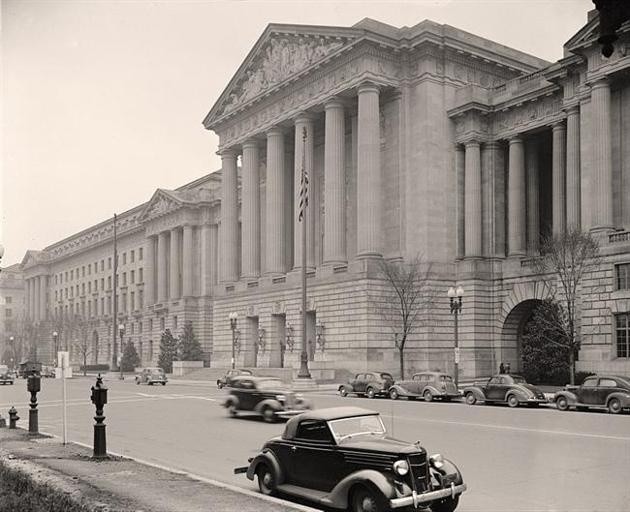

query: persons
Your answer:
[504,362,510,374]
[500,363,505,374]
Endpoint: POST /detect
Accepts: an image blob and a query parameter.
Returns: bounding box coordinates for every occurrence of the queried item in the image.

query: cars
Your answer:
[464,374,551,408]
[218,367,259,386]
[133,365,168,384]
[0,360,56,386]
[553,375,630,414]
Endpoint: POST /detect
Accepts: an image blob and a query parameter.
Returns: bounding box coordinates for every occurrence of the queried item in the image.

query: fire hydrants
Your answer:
[8,405,20,429]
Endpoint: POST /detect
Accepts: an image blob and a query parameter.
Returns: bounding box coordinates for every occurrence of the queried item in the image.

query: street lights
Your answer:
[118,323,127,382]
[448,286,466,388]
[8,335,14,360]
[226,312,237,367]
[52,331,60,369]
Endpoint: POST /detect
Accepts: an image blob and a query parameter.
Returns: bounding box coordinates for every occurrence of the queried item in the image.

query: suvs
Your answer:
[389,373,462,403]
[221,373,313,421]
[233,406,466,508]
[337,371,394,399]
